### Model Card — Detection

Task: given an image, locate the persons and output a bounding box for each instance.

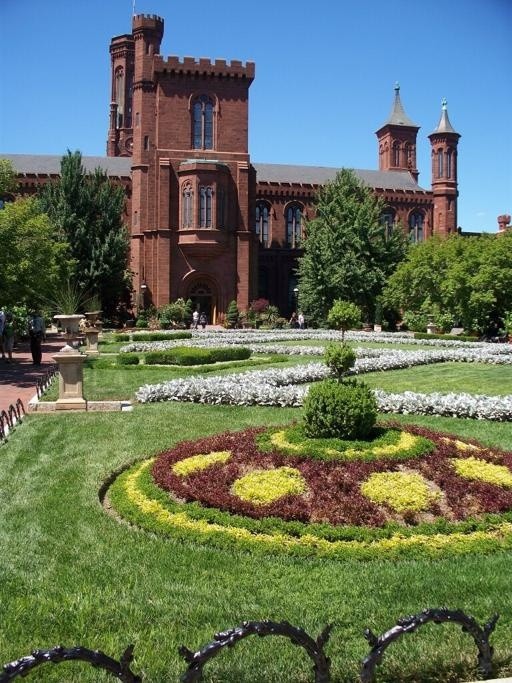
[192,309,199,329]
[200,311,208,329]
[79,317,88,328]
[0,311,7,360]
[4,310,17,363]
[297,312,305,329]
[288,312,297,328]
[28,312,46,367]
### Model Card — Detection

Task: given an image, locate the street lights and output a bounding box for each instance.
[294,287,299,322]
